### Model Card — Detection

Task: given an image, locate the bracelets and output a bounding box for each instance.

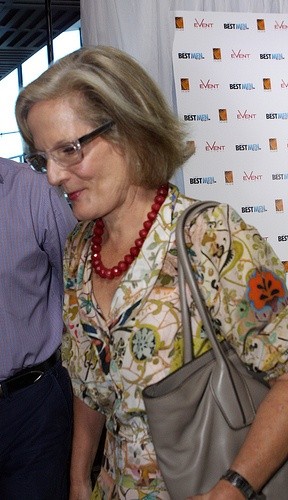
[219,469,257,500]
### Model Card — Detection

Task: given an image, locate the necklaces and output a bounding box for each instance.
[91,185,166,279]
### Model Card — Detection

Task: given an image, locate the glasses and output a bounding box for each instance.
[22,116,122,176]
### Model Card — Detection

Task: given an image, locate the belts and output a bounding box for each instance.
[0,347,63,404]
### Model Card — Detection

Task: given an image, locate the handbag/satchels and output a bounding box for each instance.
[139,196,288,500]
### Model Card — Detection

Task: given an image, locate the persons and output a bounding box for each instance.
[16,45,287,499]
[0,157,80,499]
